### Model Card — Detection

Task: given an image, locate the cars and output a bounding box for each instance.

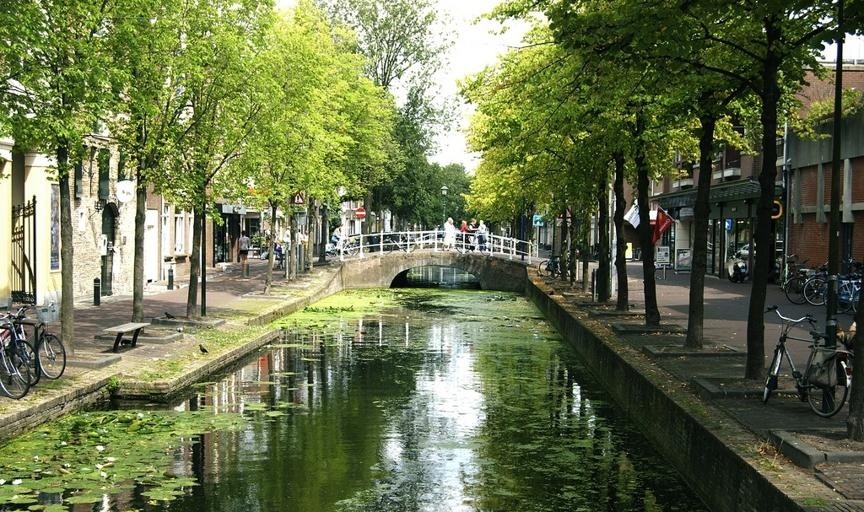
[737,244,757,257]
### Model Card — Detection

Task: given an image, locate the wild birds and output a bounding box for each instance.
[199,344,209,355]
[121,339,131,345]
[164,312,176,319]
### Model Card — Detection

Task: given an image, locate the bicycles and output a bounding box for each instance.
[781,254,861,313]
[383,234,417,252]
[762,305,855,417]
[456,236,490,255]
[539,254,570,279]
[326,238,359,257]
[0,299,66,400]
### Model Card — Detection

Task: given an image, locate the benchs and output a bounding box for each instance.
[102,322,152,353]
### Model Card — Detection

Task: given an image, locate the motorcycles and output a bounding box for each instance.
[727,251,749,283]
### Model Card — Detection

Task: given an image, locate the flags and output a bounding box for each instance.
[652,206,673,245]
[623,199,640,229]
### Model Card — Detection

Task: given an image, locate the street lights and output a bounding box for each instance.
[440,184,448,251]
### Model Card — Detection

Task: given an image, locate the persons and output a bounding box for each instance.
[266,226,346,253]
[238,231,251,267]
[441,217,489,253]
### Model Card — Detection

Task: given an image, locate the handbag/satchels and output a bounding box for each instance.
[806,348,839,389]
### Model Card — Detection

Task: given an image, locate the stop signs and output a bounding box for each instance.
[356,207,366,218]
